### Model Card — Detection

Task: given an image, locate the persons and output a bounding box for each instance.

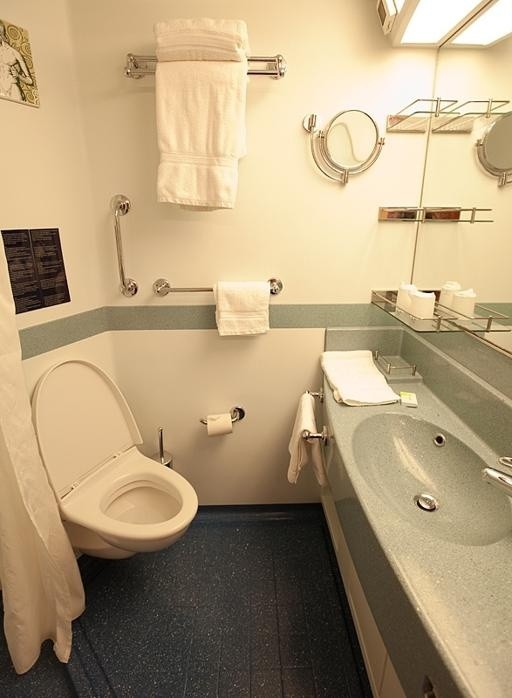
[0,20,34,104]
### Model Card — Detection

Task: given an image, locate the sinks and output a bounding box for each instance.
[353,412,512,548]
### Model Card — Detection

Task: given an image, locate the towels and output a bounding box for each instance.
[286,350,404,489]
[213,279,269,339]
[150,16,246,214]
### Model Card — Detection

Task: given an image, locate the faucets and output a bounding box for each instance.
[481,467,512,495]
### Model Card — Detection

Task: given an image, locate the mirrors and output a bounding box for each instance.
[410,0,512,360]
[320,108,381,179]
[477,110,511,187]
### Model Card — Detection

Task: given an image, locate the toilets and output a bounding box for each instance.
[32,359,198,560]
